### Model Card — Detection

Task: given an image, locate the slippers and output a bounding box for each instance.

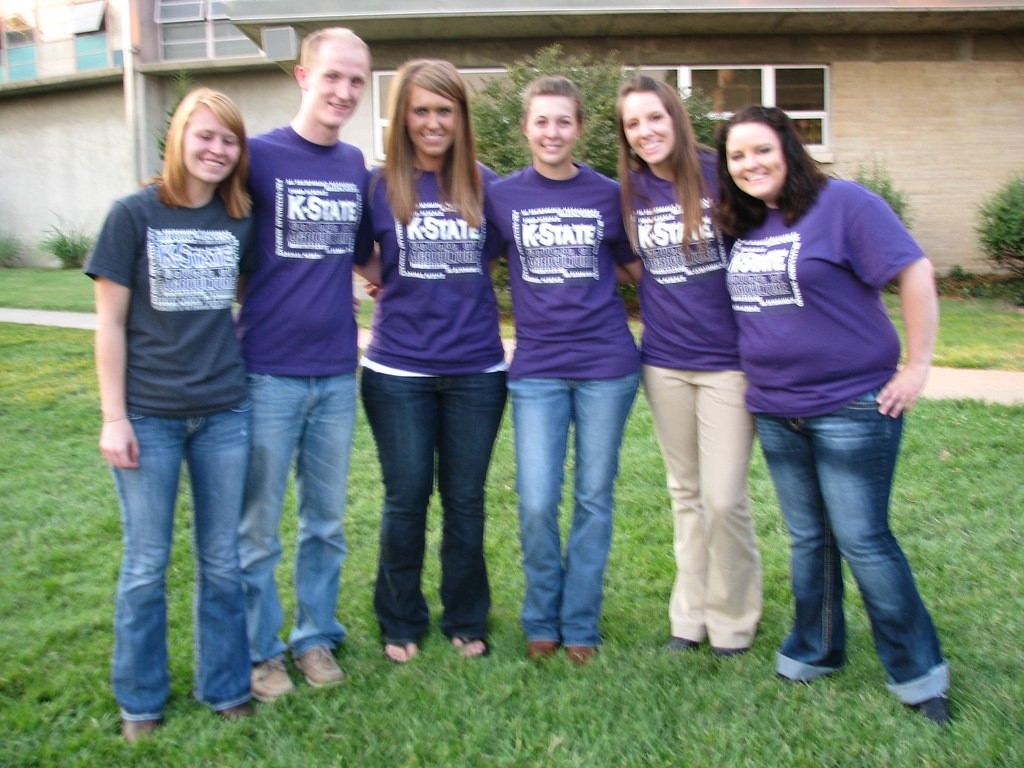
[384,641,421,664]
[449,636,490,656]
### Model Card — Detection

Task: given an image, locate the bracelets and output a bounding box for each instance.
[101,415,129,423]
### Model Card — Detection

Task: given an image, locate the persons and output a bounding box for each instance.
[236,27,385,705]
[82,86,255,741]
[614,77,766,660]
[718,104,954,728]
[358,58,509,665]
[362,75,644,668]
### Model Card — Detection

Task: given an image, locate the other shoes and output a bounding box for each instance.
[294,646,344,688]
[909,697,954,728]
[527,641,559,658]
[566,646,598,664]
[250,657,295,703]
[665,637,698,654]
[219,702,253,722]
[123,720,155,743]
[709,646,749,657]
